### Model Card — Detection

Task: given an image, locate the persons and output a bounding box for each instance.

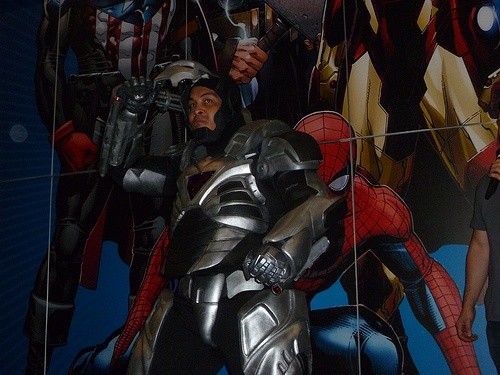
[125,75,346,375]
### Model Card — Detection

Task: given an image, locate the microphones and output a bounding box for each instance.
[485,148,500,200]
